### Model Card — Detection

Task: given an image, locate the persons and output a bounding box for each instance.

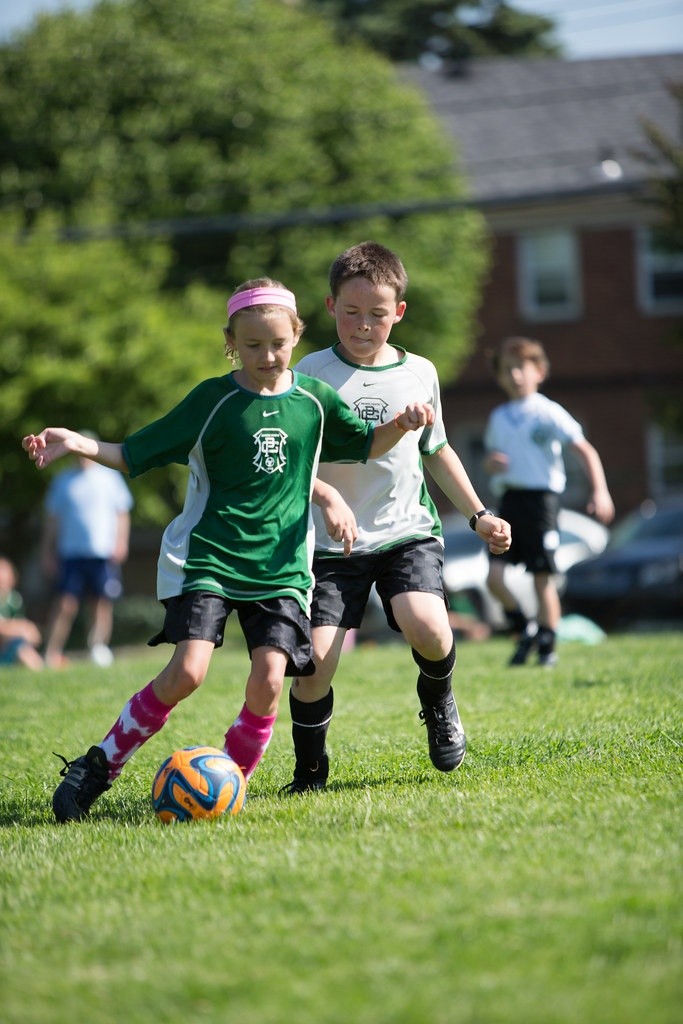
[39,430,134,666]
[476,335,615,667]
[23,279,434,827]
[0,559,46,672]
[280,240,513,800]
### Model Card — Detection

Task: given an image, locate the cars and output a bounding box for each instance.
[555,490,683,644]
[372,505,612,647]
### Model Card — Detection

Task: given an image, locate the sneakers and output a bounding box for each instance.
[416,674,466,772]
[276,751,329,798]
[52,745,113,824]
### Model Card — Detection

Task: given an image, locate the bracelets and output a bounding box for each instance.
[469,509,494,531]
[394,412,409,432]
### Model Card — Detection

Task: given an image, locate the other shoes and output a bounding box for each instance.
[535,650,558,667]
[507,638,533,665]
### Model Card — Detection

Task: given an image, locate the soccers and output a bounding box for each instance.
[151,744,248,825]
